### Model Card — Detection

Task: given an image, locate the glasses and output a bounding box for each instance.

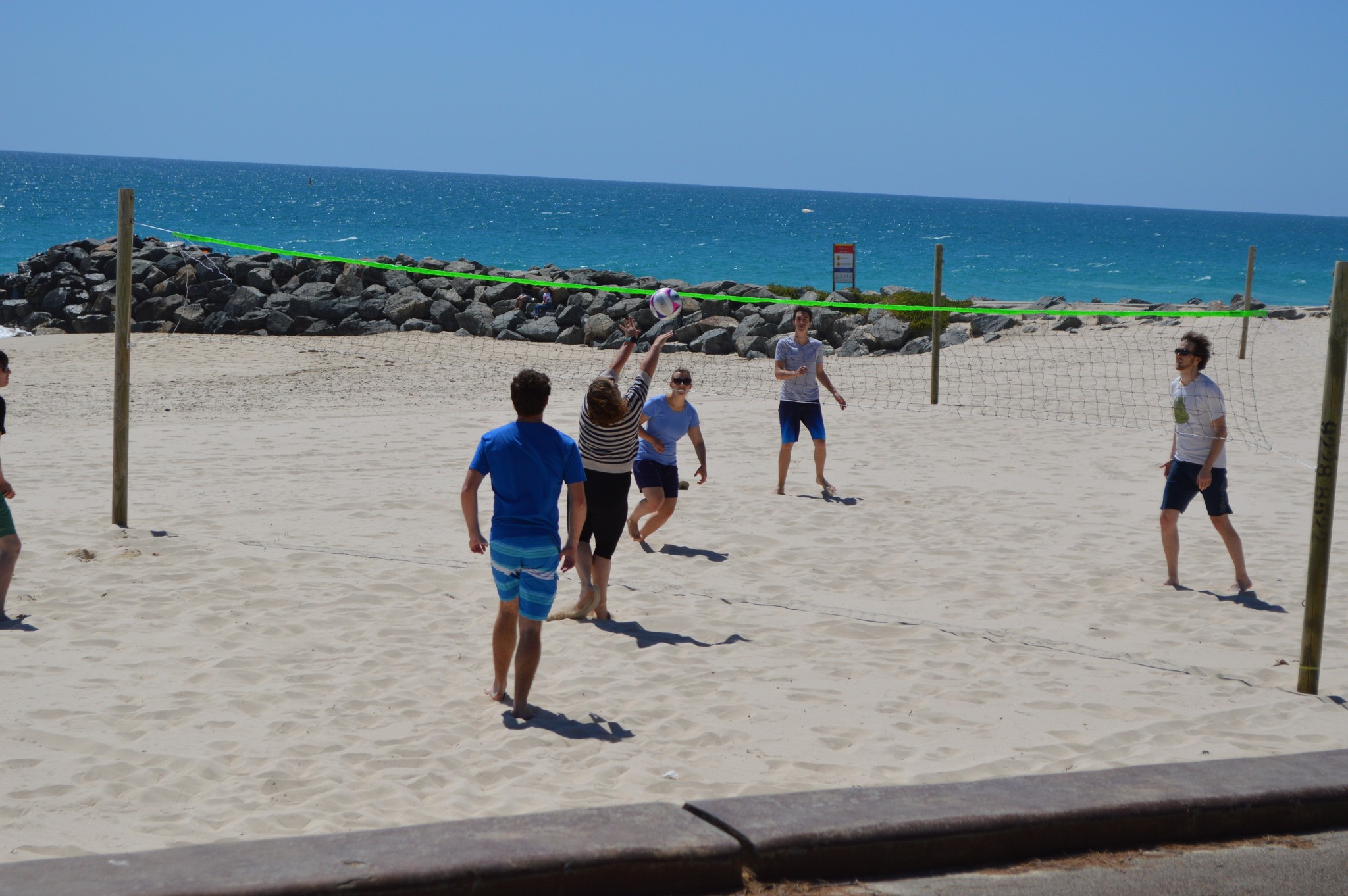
[1175,348,1201,356]
[671,378,692,385]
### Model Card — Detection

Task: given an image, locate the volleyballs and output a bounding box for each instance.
[650,288,682,320]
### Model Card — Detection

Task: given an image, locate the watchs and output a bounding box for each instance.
[625,336,637,344]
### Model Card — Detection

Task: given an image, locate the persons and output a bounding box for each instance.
[1160,331,1253,592]
[566,316,674,621]
[514,278,542,311]
[626,368,707,542]
[0,350,22,622]
[534,286,553,319]
[774,304,846,495]
[460,369,587,720]
